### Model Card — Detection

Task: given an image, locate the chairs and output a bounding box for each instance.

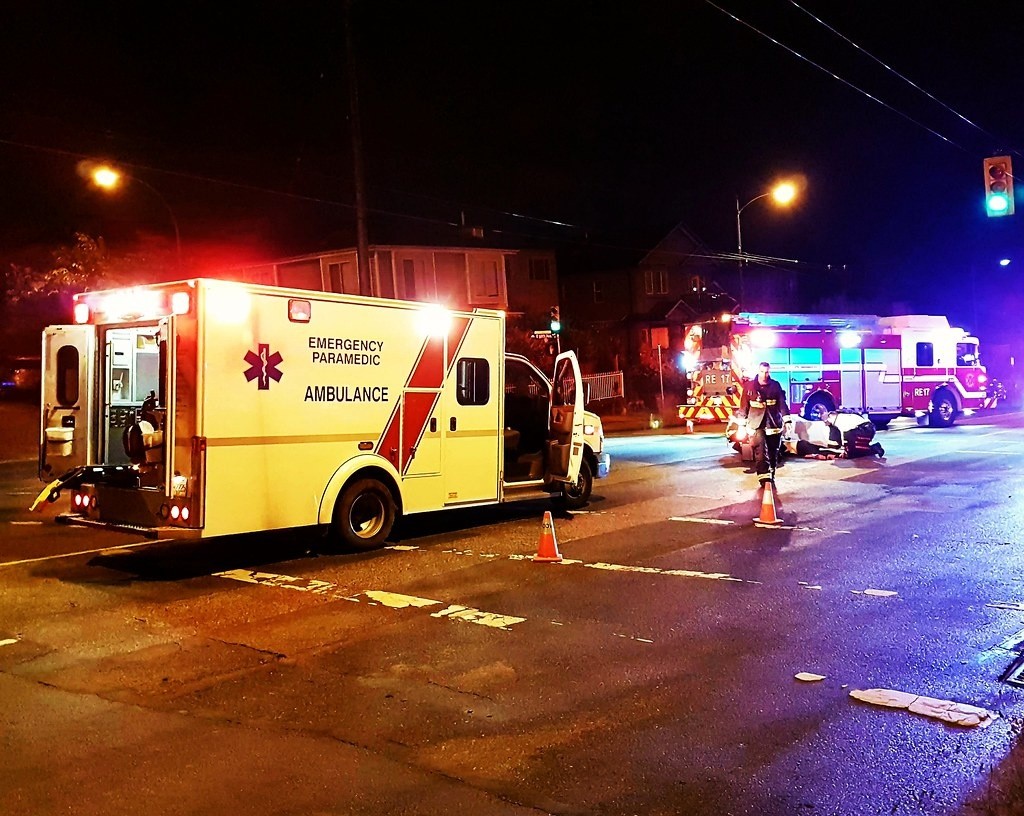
[504,428,520,449]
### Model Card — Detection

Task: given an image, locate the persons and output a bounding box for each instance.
[737,362,794,487]
[725,404,885,458]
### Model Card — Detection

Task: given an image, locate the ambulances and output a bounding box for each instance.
[30,280,611,551]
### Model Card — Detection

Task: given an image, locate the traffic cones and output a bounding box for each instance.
[532,507,565,563]
[753,482,785,526]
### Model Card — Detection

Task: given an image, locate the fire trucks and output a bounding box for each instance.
[675,311,1006,433]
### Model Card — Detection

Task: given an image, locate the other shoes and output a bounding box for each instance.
[761,480,776,490]
[873,442,885,458]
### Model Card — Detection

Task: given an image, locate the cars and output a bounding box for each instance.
[0,344,41,395]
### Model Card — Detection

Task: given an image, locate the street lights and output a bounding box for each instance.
[89,164,184,280]
[735,175,799,310]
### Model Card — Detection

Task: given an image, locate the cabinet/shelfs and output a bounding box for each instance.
[109,327,159,405]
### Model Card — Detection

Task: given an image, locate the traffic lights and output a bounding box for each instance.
[983,155,1015,217]
[548,304,562,333]
